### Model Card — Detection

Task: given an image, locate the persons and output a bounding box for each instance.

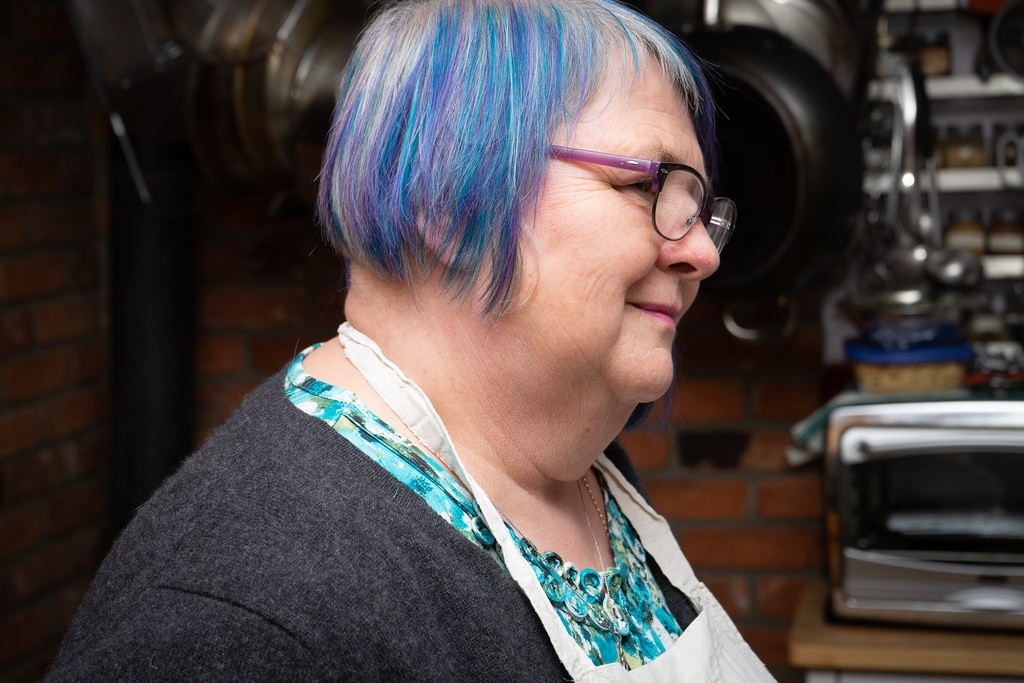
[45,1,786,679]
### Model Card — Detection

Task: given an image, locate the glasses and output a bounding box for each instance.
[542,144,737,258]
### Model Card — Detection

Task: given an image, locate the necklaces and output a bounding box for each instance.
[340,341,634,673]
